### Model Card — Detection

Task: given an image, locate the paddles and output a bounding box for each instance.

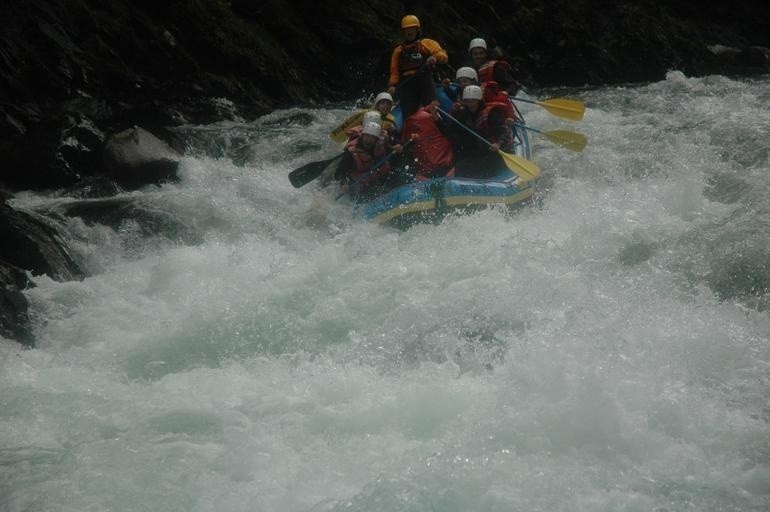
[331,65,427,144]
[435,106,539,180]
[513,121,586,152]
[288,152,345,189]
[509,95,586,120]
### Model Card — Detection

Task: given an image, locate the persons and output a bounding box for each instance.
[332,14,517,201]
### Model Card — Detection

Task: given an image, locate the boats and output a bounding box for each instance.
[353,96,540,232]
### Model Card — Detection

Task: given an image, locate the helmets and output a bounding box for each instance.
[456,67,478,82]
[462,85,482,100]
[362,122,381,138]
[400,15,421,29]
[363,111,381,123]
[375,92,393,106]
[468,38,487,53]
